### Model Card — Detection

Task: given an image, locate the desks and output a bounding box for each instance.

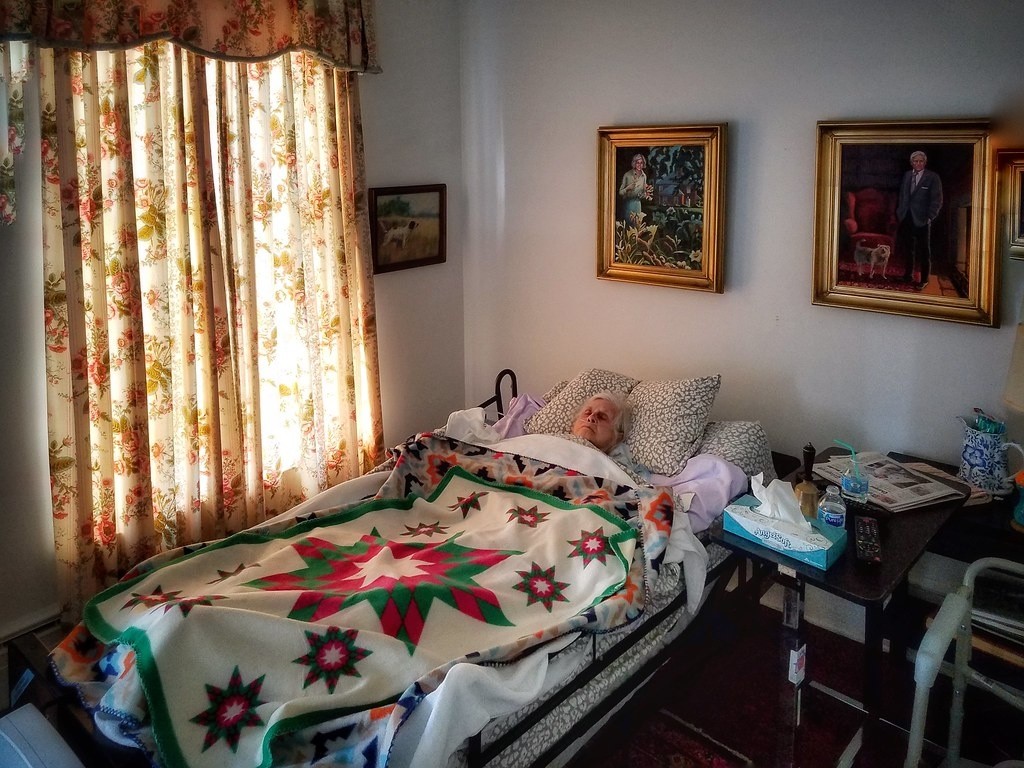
[706,444,973,751]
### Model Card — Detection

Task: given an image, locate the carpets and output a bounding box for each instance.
[584,707,754,768]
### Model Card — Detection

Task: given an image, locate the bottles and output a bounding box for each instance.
[818,485,847,529]
[841,455,870,504]
[795,472,819,519]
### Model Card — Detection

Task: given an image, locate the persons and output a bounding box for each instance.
[569,388,633,459]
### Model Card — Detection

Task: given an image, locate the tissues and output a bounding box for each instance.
[723,472,849,572]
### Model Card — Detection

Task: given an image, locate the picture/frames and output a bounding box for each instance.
[594,122,728,294]
[992,146,1024,260]
[811,117,1000,327]
[367,183,449,276]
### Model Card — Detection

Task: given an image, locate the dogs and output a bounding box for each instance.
[378,220,421,251]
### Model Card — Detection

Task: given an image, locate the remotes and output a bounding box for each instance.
[854,516,883,569]
[815,489,891,520]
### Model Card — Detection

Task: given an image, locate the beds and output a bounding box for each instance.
[7,370,802,767]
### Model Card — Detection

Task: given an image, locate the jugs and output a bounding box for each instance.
[954,415,1024,495]
